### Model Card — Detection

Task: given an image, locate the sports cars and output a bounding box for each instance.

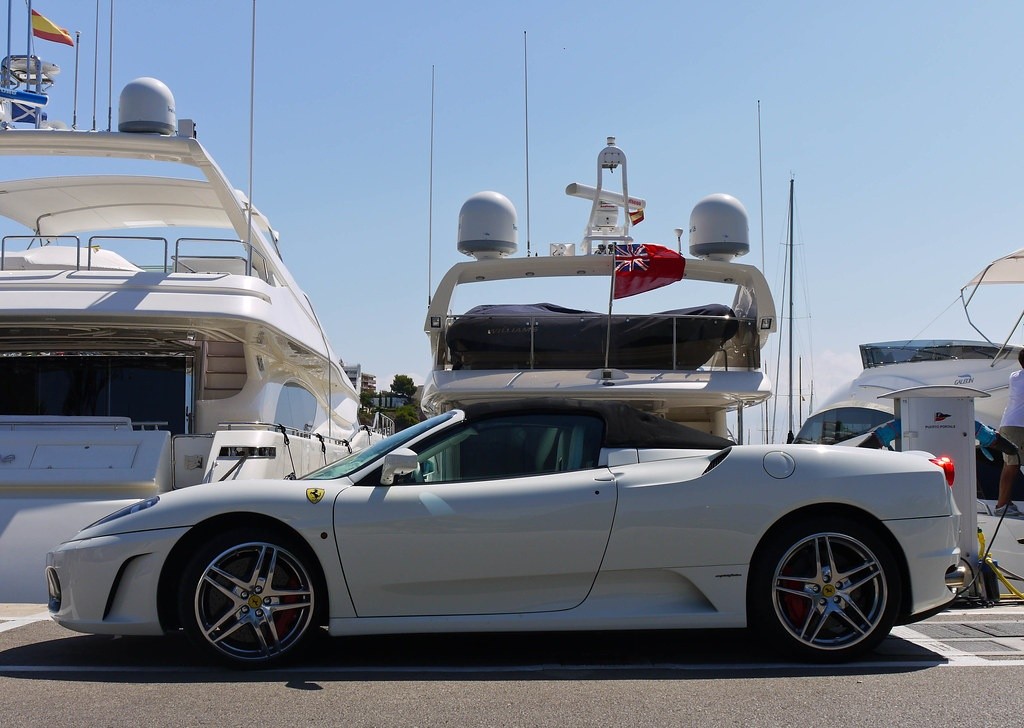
[42,399,969,682]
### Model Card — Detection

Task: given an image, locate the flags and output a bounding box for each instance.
[613,245,650,272]
[613,244,687,299]
[30,7,74,47]
[629,209,646,225]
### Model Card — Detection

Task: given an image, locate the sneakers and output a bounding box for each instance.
[994,505,1021,517]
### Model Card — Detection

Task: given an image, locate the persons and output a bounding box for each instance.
[452,349,477,372]
[992,347,1024,520]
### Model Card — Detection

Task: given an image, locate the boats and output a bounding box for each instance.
[1,1,394,602]
[414,130,785,481]
[793,243,1023,603]
